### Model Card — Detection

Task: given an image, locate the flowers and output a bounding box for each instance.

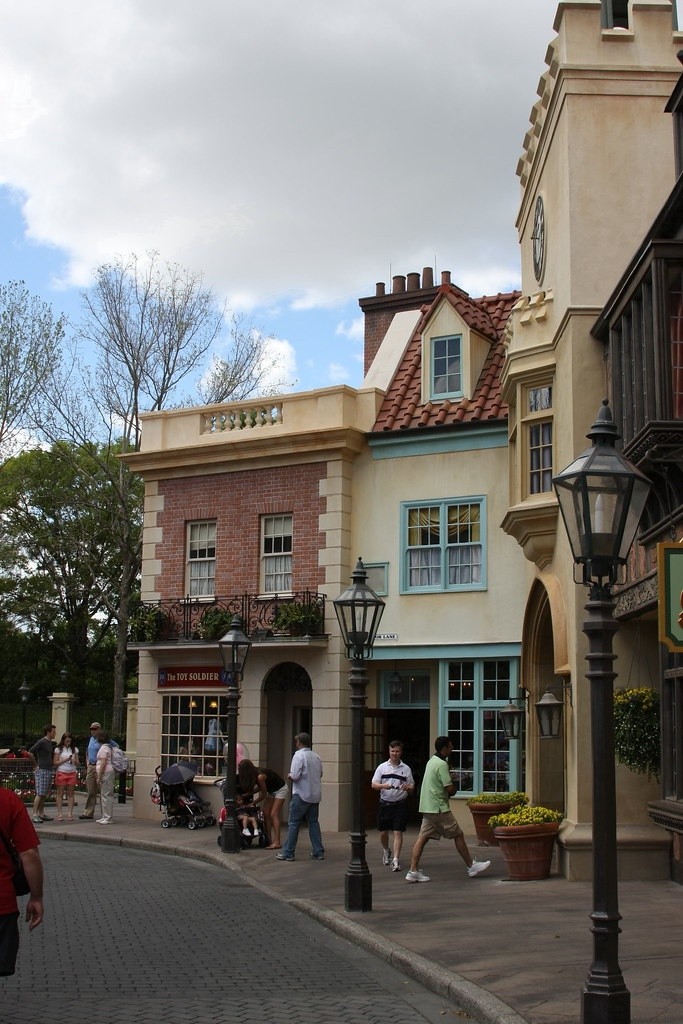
[466,793,529,805]
[613,687,662,786]
[487,803,564,830]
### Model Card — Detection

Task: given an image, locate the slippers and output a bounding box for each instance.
[265,843,282,849]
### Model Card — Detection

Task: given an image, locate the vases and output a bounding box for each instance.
[468,803,515,846]
[493,822,560,882]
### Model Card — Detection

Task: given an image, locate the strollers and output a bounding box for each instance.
[154,761,217,830]
[213,774,270,851]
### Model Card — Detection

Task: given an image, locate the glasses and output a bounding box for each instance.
[390,786,400,790]
[90,728,99,730]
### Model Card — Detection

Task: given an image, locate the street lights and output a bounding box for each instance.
[19,677,30,746]
[216,614,252,853]
[331,557,386,912]
[552,397,654,1024]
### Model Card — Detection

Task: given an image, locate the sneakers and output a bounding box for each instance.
[254,828,258,836]
[382,847,391,865]
[242,828,251,836]
[276,852,294,860]
[95,816,106,823]
[466,860,491,877]
[405,868,431,882]
[389,858,401,871]
[100,816,113,825]
[309,851,324,859]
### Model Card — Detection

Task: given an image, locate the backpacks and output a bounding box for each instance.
[103,744,129,773]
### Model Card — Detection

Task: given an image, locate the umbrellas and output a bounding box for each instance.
[160,761,198,793]
[0,748,10,755]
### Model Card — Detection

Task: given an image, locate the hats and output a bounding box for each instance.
[90,722,101,729]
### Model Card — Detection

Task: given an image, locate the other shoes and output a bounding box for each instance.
[79,814,93,819]
[39,815,54,821]
[68,815,74,821]
[32,816,43,823]
[58,815,64,821]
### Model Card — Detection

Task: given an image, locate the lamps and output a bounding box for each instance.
[533,683,572,739]
[189,700,197,708]
[209,701,218,708]
[499,687,530,740]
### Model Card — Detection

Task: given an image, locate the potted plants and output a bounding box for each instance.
[197,610,244,640]
[271,601,321,637]
[130,609,170,645]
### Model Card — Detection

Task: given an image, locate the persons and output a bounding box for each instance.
[0,746,31,772]
[54,732,79,821]
[276,733,325,861]
[28,725,56,823]
[78,722,120,825]
[238,759,289,850]
[0,786,43,977]
[236,795,259,836]
[405,736,491,883]
[371,741,415,872]
